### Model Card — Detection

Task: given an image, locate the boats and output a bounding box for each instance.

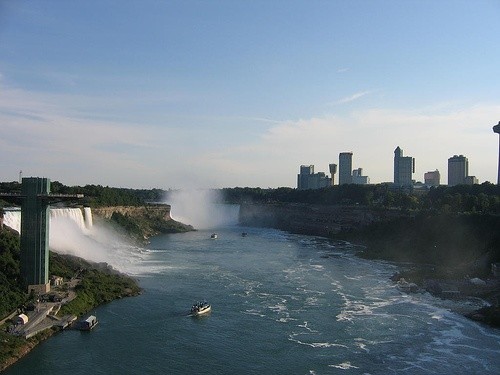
[79,316,99,331]
[191,300,211,316]
[211,233,217,240]
